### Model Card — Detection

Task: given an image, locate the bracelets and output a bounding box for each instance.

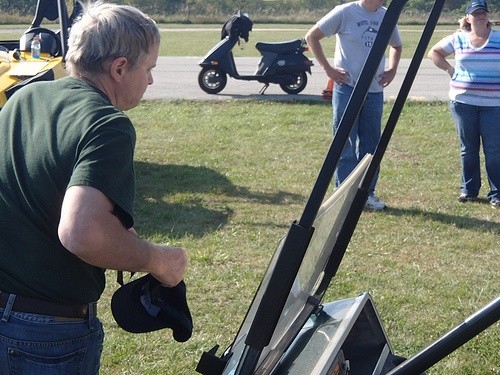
[445,67,453,72]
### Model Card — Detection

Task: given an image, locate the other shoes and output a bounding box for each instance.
[488,198,500,208]
[457,193,479,202]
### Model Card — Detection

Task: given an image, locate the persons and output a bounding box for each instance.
[305,0,403,210]
[0,0,188,375]
[428,0,500,204]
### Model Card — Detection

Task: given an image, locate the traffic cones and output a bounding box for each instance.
[322,77,334,99]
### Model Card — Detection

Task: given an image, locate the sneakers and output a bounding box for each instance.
[365,194,387,210]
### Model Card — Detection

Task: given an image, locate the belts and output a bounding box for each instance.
[0,291,99,320]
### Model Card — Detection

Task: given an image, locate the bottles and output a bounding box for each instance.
[31,36,40,61]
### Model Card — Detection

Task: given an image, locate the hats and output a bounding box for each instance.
[111,272,193,343]
[464,0,490,15]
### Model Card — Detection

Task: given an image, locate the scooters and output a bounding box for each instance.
[197,10,314,95]
